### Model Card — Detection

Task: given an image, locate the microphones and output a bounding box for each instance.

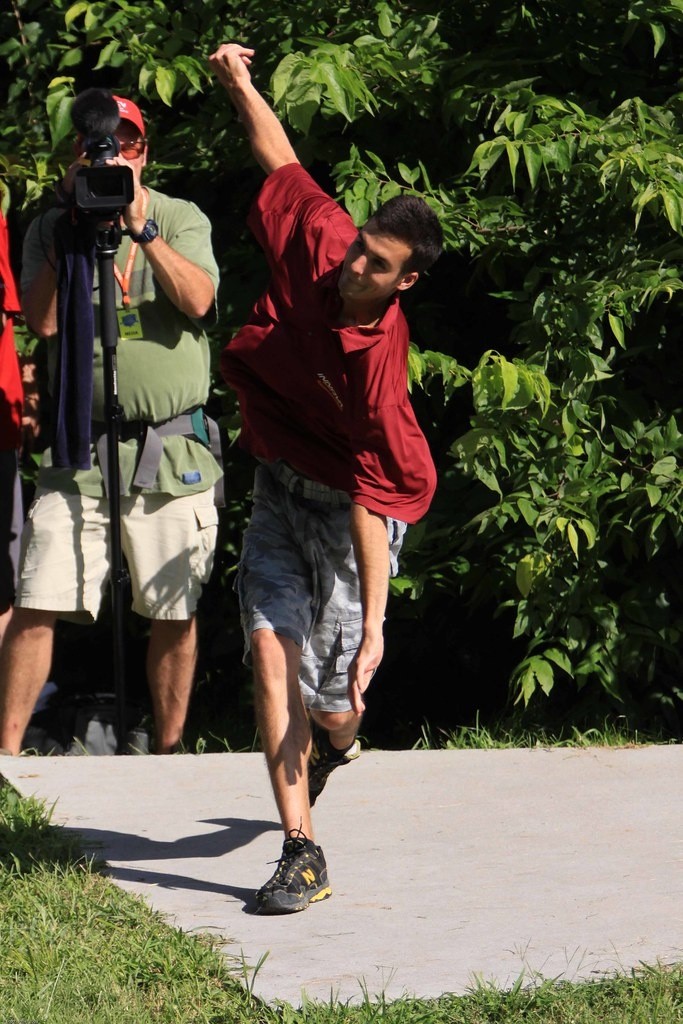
[70,87,121,140]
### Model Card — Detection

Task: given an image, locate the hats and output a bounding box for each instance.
[110,94,145,137]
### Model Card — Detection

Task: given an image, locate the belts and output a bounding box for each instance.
[270,458,353,503]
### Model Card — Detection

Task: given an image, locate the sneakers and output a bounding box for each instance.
[256,838,332,910]
[307,714,360,808]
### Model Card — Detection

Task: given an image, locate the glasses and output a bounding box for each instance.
[118,136,146,161]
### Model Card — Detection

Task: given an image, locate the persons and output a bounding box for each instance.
[204,39,437,917]
[0,84,224,760]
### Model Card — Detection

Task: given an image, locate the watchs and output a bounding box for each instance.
[131,219,158,244]
